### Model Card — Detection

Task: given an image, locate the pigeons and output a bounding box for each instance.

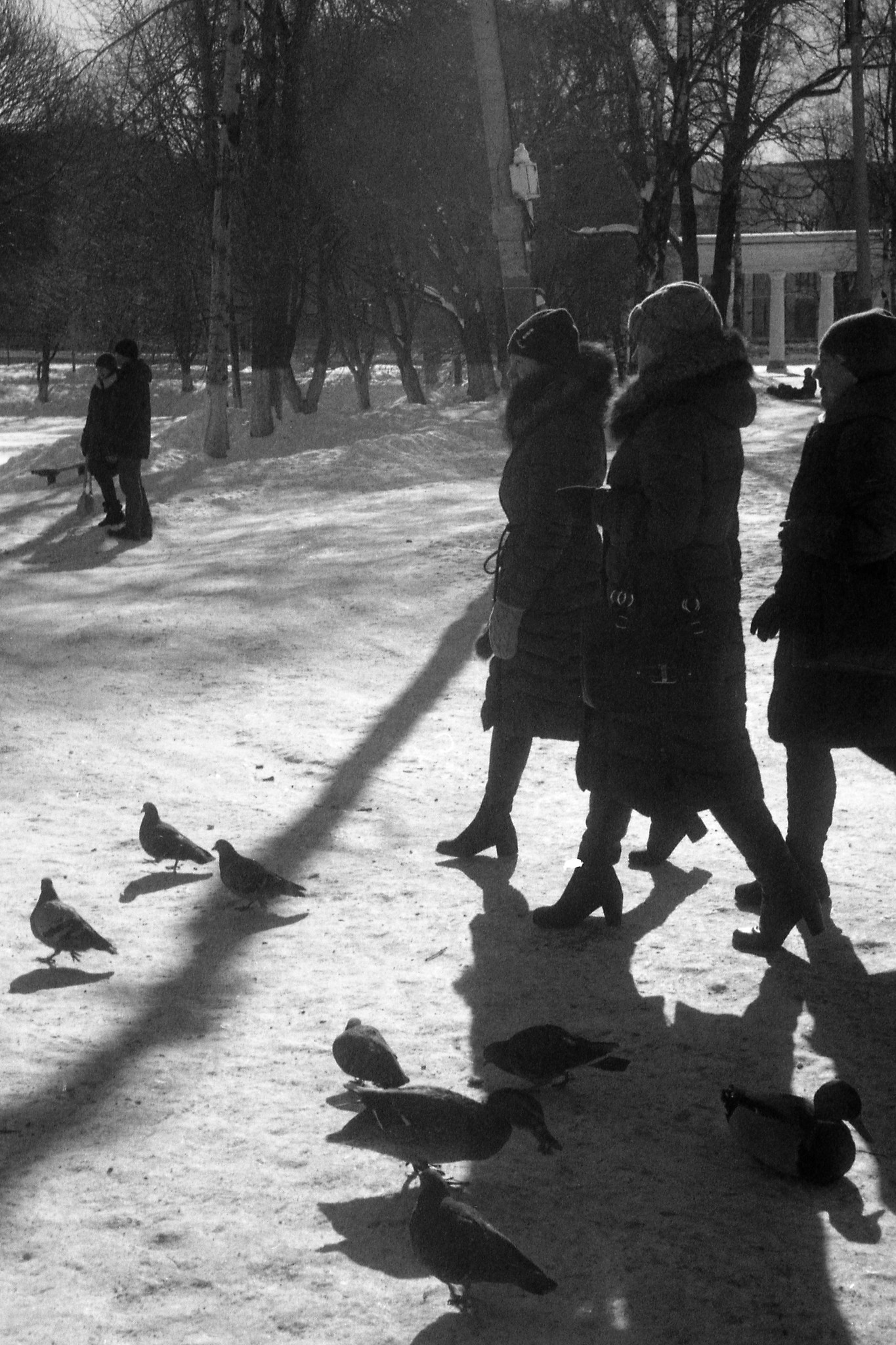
[210,839,309,910]
[409,1169,560,1307]
[31,878,120,965]
[138,801,216,872]
[479,1025,632,1091]
[334,1017,410,1090]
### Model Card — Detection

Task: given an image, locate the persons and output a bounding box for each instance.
[431,304,710,873]
[530,277,827,955]
[796,366,817,401]
[105,338,154,542]
[78,353,127,528]
[729,304,896,954]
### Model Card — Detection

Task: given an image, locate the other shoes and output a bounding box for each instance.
[108,523,153,542]
[735,854,835,903]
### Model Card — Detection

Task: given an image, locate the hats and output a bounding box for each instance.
[817,301,895,384]
[115,339,139,359]
[505,307,579,365]
[627,280,722,356]
[95,354,118,373]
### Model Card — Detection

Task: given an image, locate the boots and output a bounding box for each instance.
[98,501,124,527]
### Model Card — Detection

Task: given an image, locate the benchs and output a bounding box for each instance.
[32,458,88,486]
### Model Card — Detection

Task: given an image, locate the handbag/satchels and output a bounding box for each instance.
[76,491,95,517]
[575,612,713,719]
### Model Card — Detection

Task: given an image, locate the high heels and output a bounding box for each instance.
[628,808,709,867]
[531,866,623,927]
[730,874,826,954]
[433,813,518,860]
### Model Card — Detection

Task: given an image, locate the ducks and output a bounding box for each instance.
[323,1083,564,1185]
[716,1080,876,1189]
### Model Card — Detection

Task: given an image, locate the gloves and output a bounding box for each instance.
[778,514,834,559]
[749,595,788,644]
[555,484,595,520]
[486,600,526,659]
